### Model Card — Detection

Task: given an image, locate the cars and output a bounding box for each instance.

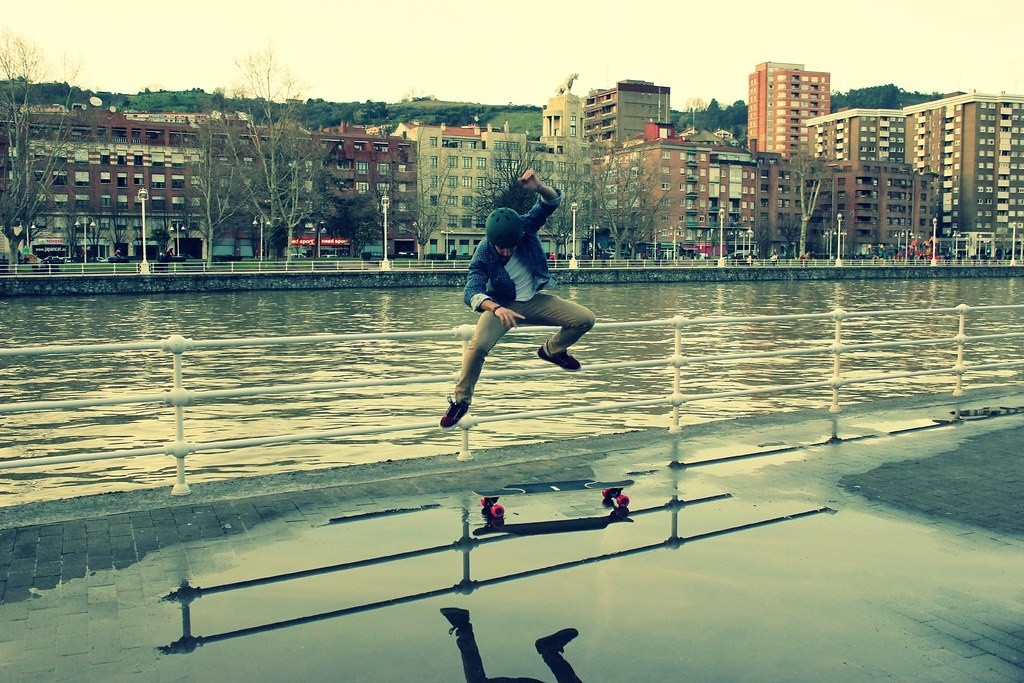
[90,257,107,263]
[291,254,306,258]
[42,255,66,264]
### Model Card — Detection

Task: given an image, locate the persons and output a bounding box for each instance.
[800,255,805,266]
[439,167,595,432]
[166,247,174,260]
[18,250,22,264]
[600,251,607,267]
[772,254,777,267]
[115,248,122,258]
[440,608,583,683]
[550,252,555,268]
[919,253,939,264]
[32,254,38,272]
[909,253,913,264]
[944,252,1001,266]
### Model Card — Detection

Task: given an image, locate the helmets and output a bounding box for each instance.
[485,207,525,245]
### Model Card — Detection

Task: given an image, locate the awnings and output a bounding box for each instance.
[681,244,698,250]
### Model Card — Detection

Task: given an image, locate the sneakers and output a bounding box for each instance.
[440,396,470,431]
[537,345,581,372]
[440,607,470,634]
[535,628,578,654]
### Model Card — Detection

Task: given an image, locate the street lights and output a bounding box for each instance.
[252,214,271,262]
[380,186,390,271]
[841,228,848,260]
[169,220,186,257]
[952,231,961,260]
[441,230,455,260]
[894,230,905,261]
[825,227,837,262]
[310,221,324,258]
[718,207,726,268]
[137,188,150,275]
[746,226,753,258]
[74,214,96,264]
[568,202,579,269]
[729,229,742,266]
[911,232,922,265]
[589,223,600,267]
[19,220,36,263]
[978,233,982,260]
[836,212,843,268]
[901,228,914,262]
[649,227,662,260]
[929,217,938,266]
[669,221,683,264]
[965,235,970,260]
[1008,222,1018,265]
[740,231,749,259]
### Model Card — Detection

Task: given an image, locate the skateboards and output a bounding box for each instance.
[473,478,635,517]
[472,508,635,537]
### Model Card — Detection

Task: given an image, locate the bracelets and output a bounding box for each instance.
[493,306,502,317]
